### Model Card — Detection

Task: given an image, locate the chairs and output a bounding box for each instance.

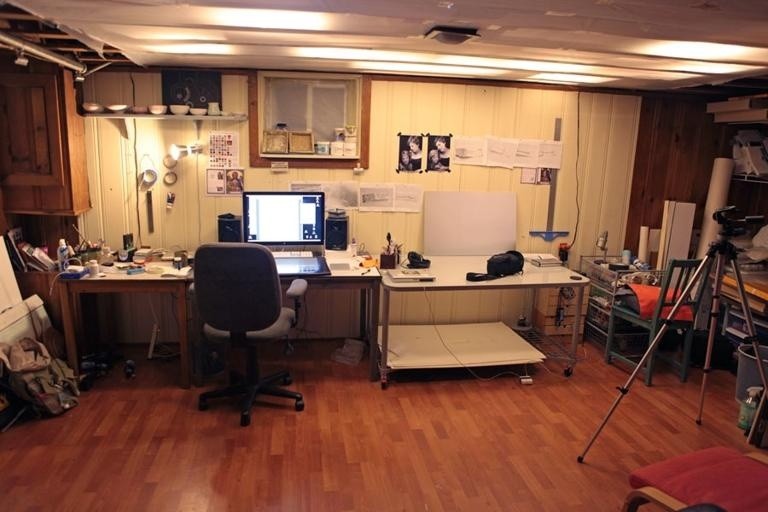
[619,448,768,512]
[188,241,309,425]
[603,257,709,391]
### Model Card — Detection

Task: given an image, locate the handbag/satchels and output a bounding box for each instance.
[465,249,524,283]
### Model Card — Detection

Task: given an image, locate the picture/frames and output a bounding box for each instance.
[287,130,315,154]
[261,129,288,154]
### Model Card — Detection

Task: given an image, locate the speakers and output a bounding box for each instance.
[326,216,349,250]
[218,216,243,242]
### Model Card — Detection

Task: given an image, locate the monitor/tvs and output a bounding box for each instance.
[242,191,326,246]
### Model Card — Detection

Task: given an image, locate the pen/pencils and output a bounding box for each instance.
[361,269,371,276]
[385,244,404,255]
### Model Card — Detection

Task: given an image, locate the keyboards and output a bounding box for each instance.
[271,251,314,258]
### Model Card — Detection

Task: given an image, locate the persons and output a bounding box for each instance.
[428,149,439,170]
[428,136,449,170]
[408,136,422,171]
[399,150,412,170]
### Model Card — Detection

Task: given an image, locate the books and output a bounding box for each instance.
[387,269,436,284]
[4,228,55,273]
[531,257,562,267]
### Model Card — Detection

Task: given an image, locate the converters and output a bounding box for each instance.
[519,375,533,385]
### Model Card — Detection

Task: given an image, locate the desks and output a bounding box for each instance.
[188,249,382,384]
[56,245,193,390]
[370,253,592,389]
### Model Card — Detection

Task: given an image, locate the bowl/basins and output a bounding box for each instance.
[82,102,245,117]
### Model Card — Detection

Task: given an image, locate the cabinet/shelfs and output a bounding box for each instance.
[577,253,670,354]
[1,68,93,216]
[532,268,587,336]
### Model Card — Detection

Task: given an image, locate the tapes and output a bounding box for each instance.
[140,169,158,186]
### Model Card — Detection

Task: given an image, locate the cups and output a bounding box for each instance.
[175,251,188,266]
[132,256,146,264]
[118,250,128,262]
[71,246,101,265]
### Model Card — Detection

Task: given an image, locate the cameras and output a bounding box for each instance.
[711,203,739,226]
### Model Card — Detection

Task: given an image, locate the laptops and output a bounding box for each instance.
[274,257,332,276]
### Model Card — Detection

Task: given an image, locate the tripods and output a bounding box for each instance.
[576,235,768,463]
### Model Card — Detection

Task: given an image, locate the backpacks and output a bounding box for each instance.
[0,336,80,417]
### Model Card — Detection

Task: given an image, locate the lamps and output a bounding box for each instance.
[166,120,204,161]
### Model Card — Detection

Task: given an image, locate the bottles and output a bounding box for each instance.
[173,257,182,269]
[101,247,114,266]
[351,238,356,258]
[89,258,100,277]
[620,249,644,271]
[57,238,68,273]
[275,123,288,131]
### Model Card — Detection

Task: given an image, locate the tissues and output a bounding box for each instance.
[379,239,398,270]
